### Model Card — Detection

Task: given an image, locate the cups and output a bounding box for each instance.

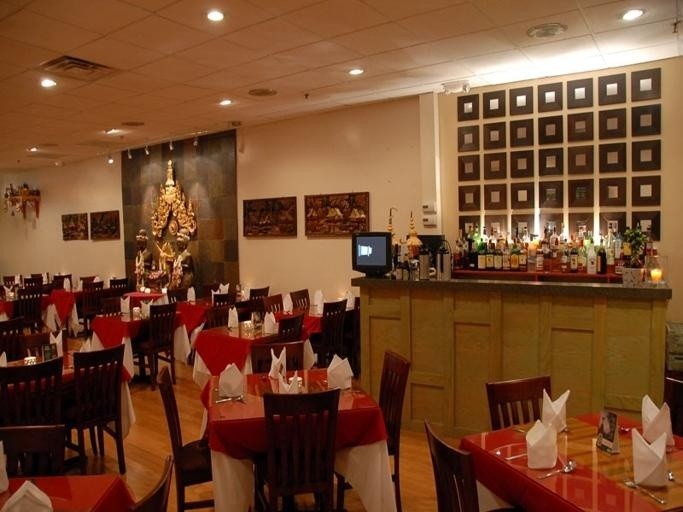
[243,321,251,330]
[24,356,37,366]
[133,307,141,315]
[9,293,14,299]
[644,255,668,285]
[290,376,303,388]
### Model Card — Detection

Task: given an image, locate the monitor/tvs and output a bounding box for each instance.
[351,231,392,278]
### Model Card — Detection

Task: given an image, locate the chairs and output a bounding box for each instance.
[424,420,520,511]
[243,389,334,511]
[130,456,174,512]
[0,272,361,390]
[335,350,410,511]
[1,344,136,476]
[484,375,552,431]
[158,365,214,511]
[664,377,681,437]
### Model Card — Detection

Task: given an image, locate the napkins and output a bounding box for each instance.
[526,419,558,469]
[540,387,570,434]
[2,479,53,511]
[269,347,286,378]
[328,354,353,389]
[639,394,675,451]
[218,362,243,397]
[632,428,669,489]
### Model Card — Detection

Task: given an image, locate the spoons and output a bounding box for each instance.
[502,424,679,507]
[215,395,243,404]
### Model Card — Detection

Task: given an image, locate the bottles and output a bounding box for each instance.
[391,239,449,280]
[622,226,658,269]
[452,224,625,276]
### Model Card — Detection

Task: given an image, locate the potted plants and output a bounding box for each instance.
[616,220,650,284]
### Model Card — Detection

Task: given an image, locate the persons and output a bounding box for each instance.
[162,235,195,297]
[135,230,152,291]
